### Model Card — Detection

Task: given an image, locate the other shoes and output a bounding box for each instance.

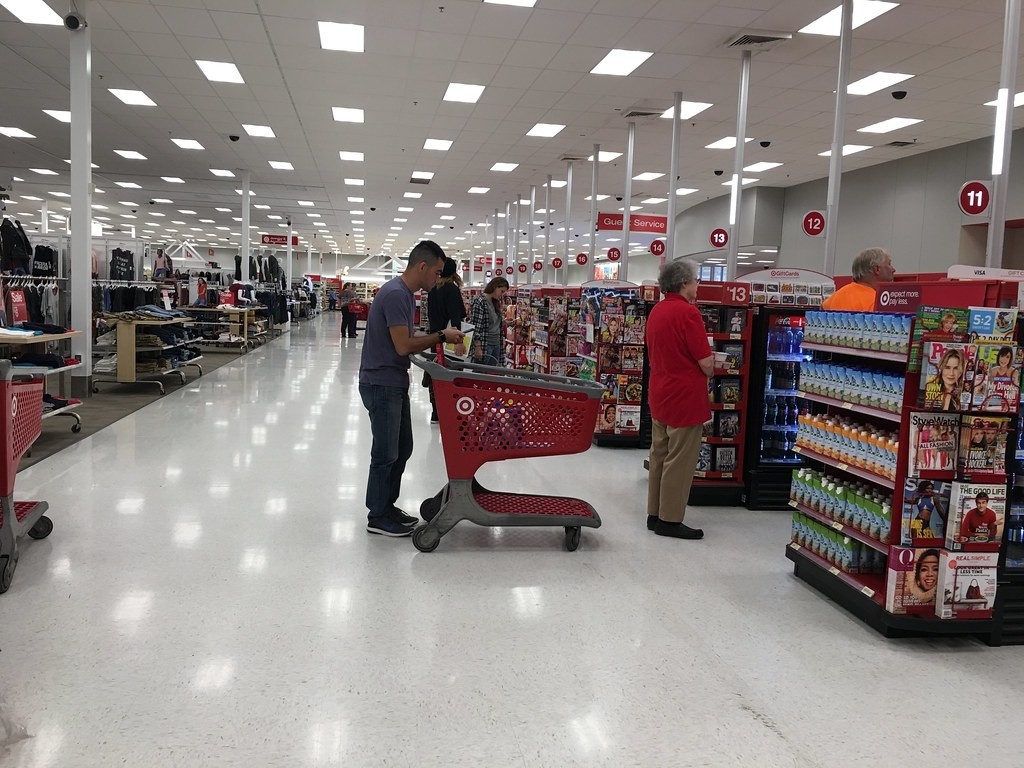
[342,335,346,337]
[431,412,438,423]
[349,335,356,338]
[366,510,415,537]
[655,518,704,539]
[647,515,658,529]
[387,506,418,526]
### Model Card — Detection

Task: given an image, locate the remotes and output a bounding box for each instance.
[346,234,349,236]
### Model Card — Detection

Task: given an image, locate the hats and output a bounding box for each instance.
[440,257,456,277]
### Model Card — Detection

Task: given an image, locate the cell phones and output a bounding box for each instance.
[512,317,520,322]
[462,328,476,334]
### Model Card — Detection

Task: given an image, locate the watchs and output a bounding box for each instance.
[437,331,446,342]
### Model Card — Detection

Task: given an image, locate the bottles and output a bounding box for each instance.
[761,325,802,460]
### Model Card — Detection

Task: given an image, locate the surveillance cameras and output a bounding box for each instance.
[62,12,86,33]
[892,91,907,100]
[286,220,291,226]
[132,210,136,213]
[367,248,370,250]
[371,208,375,211]
[229,136,240,141]
[450,226,454,229]
[616,197,622,201]
[760,141,770,147]
[714,170,723,175]
[523,223,579,238]
[149,201,154,205]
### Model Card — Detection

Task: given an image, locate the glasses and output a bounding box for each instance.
[689,279,701,284]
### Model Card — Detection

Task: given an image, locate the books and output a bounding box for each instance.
[886,306,1024,619]
[503,297,645,436]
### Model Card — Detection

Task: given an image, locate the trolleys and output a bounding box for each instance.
[347,298,371,330]
[0,358,54,593]
[408,349,608,553]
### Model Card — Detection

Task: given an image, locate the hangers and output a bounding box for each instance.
[92,279,157,292]
[0,274,58,290]
[119,243,127,252]
[41,238,51,246]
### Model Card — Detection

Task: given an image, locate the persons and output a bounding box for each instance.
[991,345,1018,394]
[471,277,508,375]
[932,313,957,334]
[421,257,467,425]
[971,422,986,451]
[608,318,619,343]
[365,240,466,537]
[645,261,715,540]
[911,549,939,603]
[338,282,361,338]
[986,421,998,447]
[904,481,945,536]
[960,492,1003,538]
[821,248,896,312]
[938,348,965,410]
[329,288,336,312]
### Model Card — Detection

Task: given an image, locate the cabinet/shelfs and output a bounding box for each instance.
[157,276,323,354]
[356,281,659,449]
[92,315,203,398]
[784,278,1023,647]
[643,280,835,511]
[0,331,84,457]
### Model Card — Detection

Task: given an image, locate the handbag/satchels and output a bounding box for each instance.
[348,300,362,313]
[966,579,980,599]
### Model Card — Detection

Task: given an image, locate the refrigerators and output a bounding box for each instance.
[740,304,820,511]
